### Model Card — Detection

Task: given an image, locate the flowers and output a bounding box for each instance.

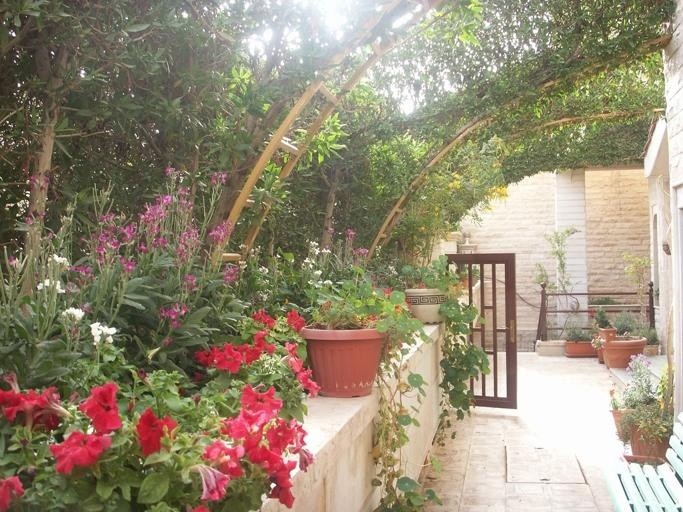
[609,351,674,474]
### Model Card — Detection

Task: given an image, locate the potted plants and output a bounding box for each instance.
[536,225,660,365]
